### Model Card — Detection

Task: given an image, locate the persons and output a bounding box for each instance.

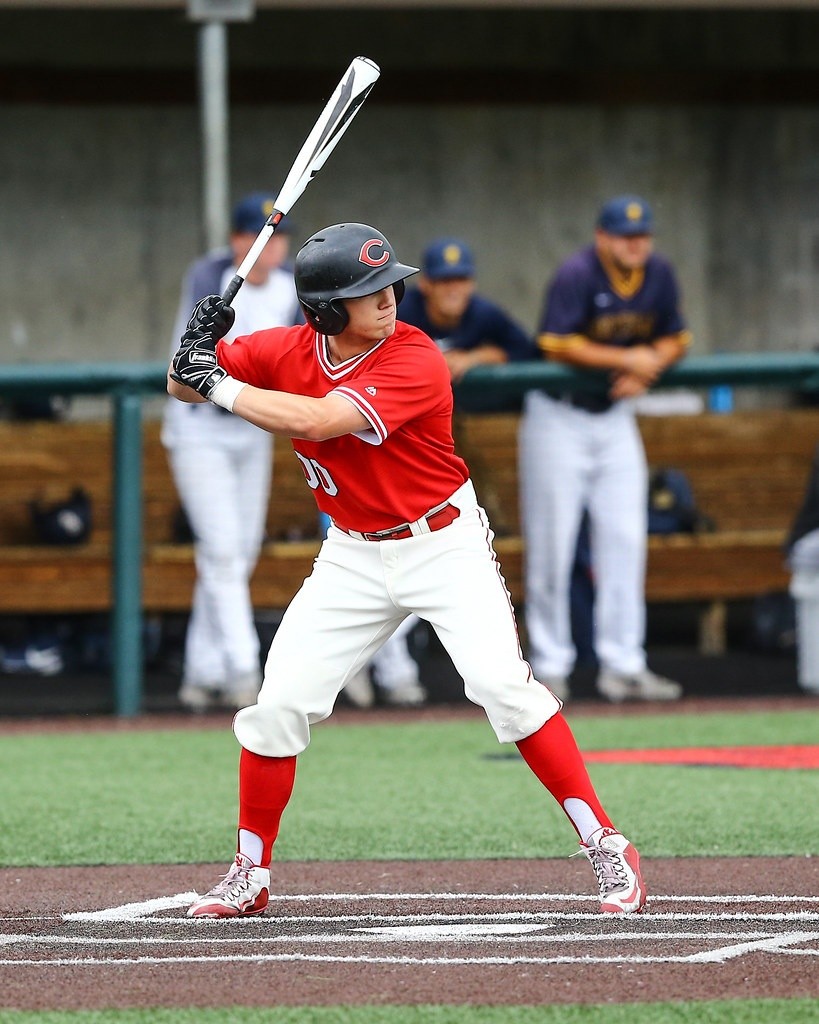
[160,192,306,710]
[166,222,646,919]
[518,192,685,703]
[342,237,537,710]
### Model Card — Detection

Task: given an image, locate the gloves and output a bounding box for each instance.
[185,294,235,345]
[170,330,228,399]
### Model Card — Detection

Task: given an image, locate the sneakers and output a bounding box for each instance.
[187,853,270,918]
[569,826,647,915]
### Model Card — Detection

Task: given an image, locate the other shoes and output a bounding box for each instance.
[221,672,262,708]
[390,680,425,703]
[342,666,373,706]
[542,681,568,702]
[180,686,208,706]
[599,667,680,700]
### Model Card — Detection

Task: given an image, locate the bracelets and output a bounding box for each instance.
[209,374,249,414]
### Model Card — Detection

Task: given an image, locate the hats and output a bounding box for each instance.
[231,193,288,234]
[294,222,420,336]
[422,239,474,278]
[599,196,651,235]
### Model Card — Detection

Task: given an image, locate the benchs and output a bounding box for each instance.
[0,410,818,663]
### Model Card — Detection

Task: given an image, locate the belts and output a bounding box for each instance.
[333,503,460,542]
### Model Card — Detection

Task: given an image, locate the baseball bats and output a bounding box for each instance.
[168,52,384,387]
